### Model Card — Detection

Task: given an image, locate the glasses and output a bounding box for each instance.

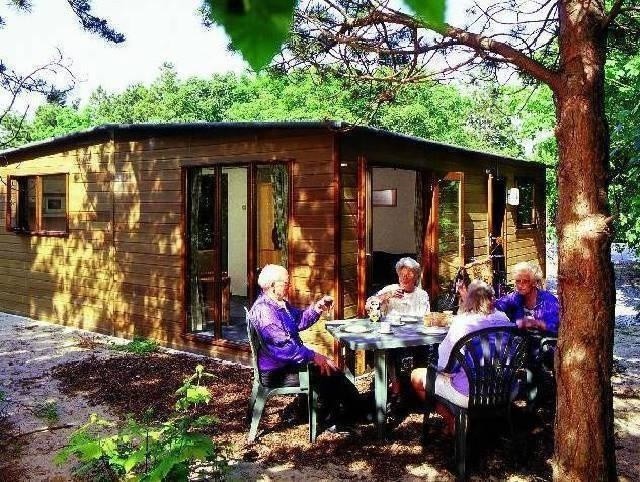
[398,273,415,278]
[516,279,531,284]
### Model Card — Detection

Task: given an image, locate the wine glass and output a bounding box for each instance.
[397,289,404,303]
[324,296,333,316]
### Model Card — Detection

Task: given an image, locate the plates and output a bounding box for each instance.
[340,310,456,335]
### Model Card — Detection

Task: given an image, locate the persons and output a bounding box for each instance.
[410,280,518,437]
[457,265,559,400]
[247,263,393,424]
[365,257,431,404]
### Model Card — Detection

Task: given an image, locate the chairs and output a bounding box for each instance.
[242,306,321,445]
[533,336,555,400]
[423,327,534,475]
